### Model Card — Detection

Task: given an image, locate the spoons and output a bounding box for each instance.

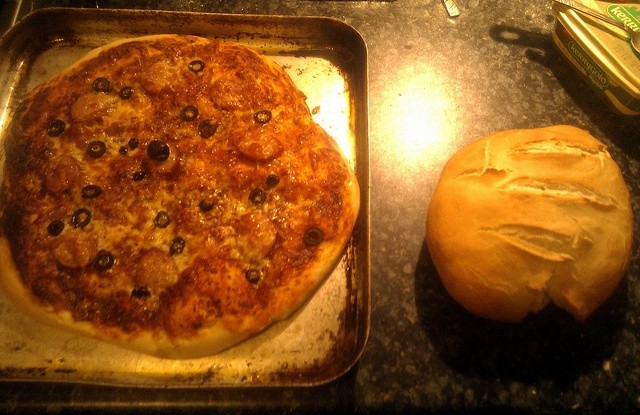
[553,0,640,54]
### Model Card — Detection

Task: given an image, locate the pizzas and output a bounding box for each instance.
[1,34,361,360]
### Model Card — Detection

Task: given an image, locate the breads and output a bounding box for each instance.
[426,125,634,322]
